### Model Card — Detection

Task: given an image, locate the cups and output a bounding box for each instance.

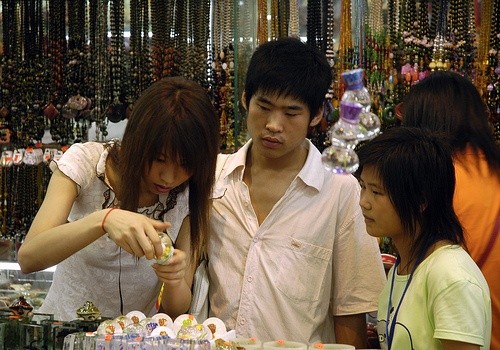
[262,340,307,350]
[309,343,356,349]
[223,337,262,350]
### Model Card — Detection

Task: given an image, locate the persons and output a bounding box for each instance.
[357,126,492,350]
[207,33,386,349]
[17,74,218,323]
[395,69,500,350]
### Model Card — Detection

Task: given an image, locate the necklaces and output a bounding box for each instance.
[0,0,499,139]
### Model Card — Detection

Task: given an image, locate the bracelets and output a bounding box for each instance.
[101,205,116,234]
